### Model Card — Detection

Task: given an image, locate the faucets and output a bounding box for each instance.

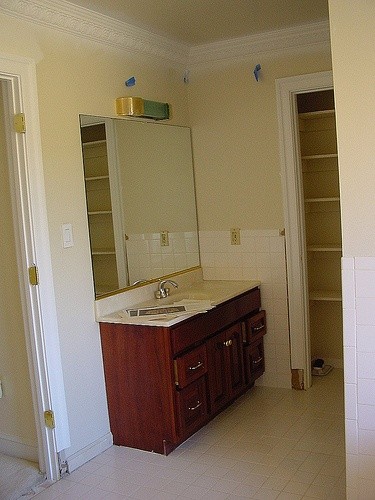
[156,279,178,299]
[130,278,149,285]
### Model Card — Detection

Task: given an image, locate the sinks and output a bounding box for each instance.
[164,299,212,314]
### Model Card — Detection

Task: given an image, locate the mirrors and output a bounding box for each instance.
[77,113,201,300]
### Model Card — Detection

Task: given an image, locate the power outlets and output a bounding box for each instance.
[160,230,168,246]
[230,228,240,245]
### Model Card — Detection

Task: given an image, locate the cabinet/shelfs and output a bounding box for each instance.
[100,312,207,456]
[79,118,129,297]
[241,287,267,390]
[299,111,344,371]
[206,302,247,421]
